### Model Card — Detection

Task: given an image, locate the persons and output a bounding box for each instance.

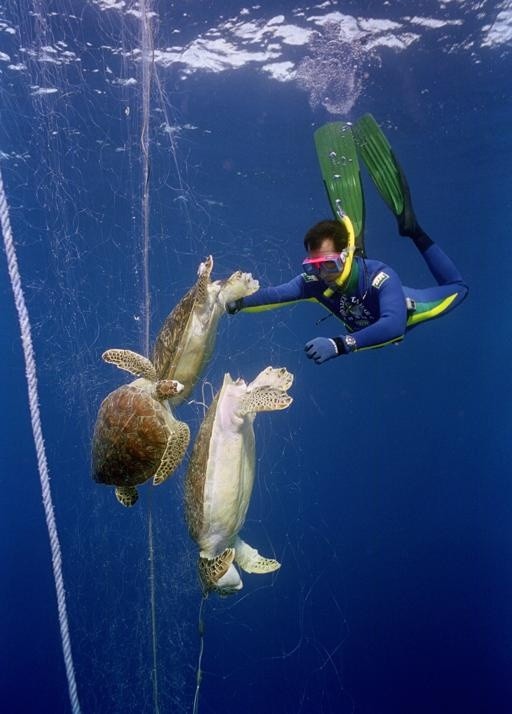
[225,112,470,364]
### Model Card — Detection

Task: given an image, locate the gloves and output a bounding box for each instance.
[302,337,340,364]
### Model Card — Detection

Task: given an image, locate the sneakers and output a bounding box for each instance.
[398,200,413,237]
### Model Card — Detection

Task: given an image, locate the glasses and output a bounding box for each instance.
[302,255,345,276]
